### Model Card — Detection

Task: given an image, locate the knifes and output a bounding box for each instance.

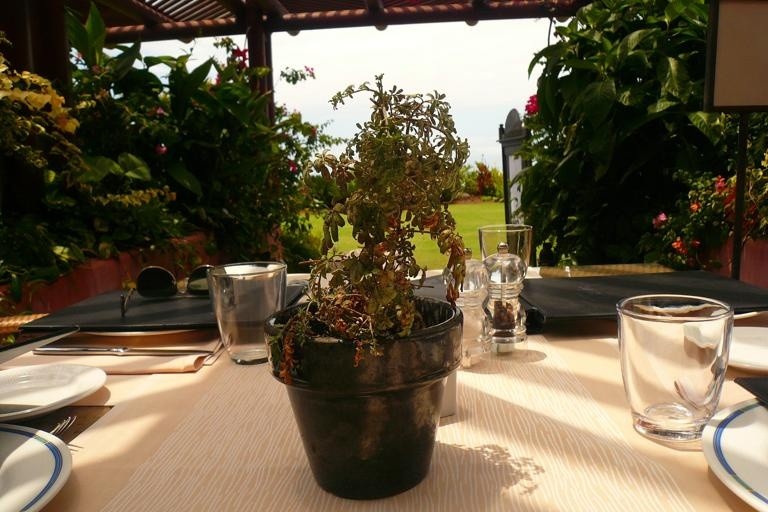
[29,346,215,358]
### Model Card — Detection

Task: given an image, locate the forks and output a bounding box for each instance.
[45,414,80,438]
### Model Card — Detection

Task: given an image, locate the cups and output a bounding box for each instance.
[613,289,736,449]
[477,223,535,260]
[205,260,288,365]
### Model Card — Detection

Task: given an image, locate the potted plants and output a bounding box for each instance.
[262,70,473,502]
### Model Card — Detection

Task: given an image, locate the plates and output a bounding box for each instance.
[721,312,767,374]
[0,362,107,424]
[699,395,768,510]
[0,423,73,511]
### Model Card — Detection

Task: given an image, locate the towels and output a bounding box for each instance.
[0,331,235,375]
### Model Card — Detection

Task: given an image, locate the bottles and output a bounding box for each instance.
[457,241,532,370]
